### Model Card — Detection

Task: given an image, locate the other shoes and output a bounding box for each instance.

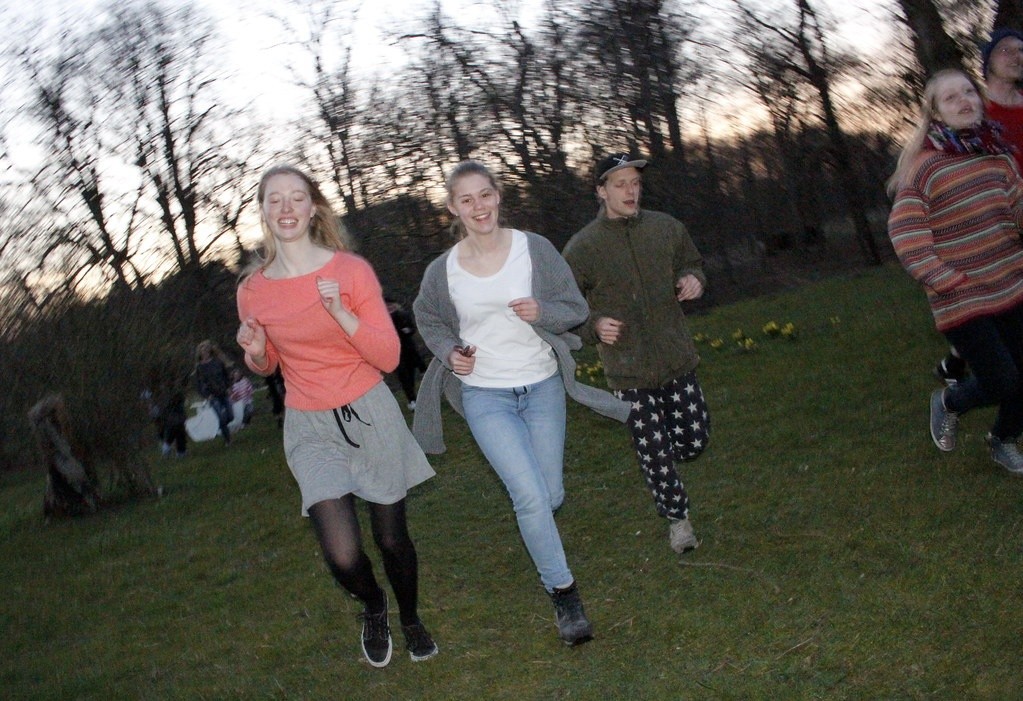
[408,400,416,410]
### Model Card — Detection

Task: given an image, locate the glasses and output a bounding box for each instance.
[994,46,1023,56]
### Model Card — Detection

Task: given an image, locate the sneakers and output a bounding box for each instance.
[361,587,394,667]
[670,520,697,553]
[546,581,596,647]
[930,388,959,451]
[988,432,1023,471]
[401,620,437,662]
[931,358,961,391]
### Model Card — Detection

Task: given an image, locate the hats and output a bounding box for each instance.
[595,152,647,186]
[983,29,1023,73]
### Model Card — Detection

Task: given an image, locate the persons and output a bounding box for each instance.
[411,161,632,648]
[235,166,440,670]
[932,26,1023,388]
[28,334,284,526]
[562,152,712,551]
[889,67,1023,472]
[385,296,428,414]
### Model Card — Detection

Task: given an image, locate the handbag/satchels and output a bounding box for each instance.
[184,405,219,441]
